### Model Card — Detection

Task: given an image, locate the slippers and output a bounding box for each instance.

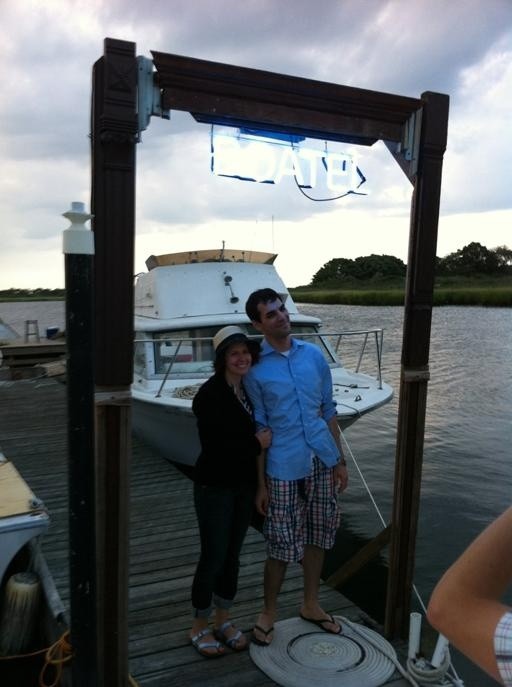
[300,612,342,634]
[251,624,274,646]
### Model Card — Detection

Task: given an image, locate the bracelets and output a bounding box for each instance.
[338,456,347,466]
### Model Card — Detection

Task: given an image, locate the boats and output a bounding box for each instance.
[129,248,393,478]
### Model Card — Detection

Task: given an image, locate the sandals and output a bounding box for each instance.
[191,622,248,658]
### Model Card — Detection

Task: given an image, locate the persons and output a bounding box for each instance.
[425,503,512,686]
[238,286,348,645]
[187,325,273,658]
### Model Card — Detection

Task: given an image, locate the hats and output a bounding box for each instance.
[212,325,260,357]
